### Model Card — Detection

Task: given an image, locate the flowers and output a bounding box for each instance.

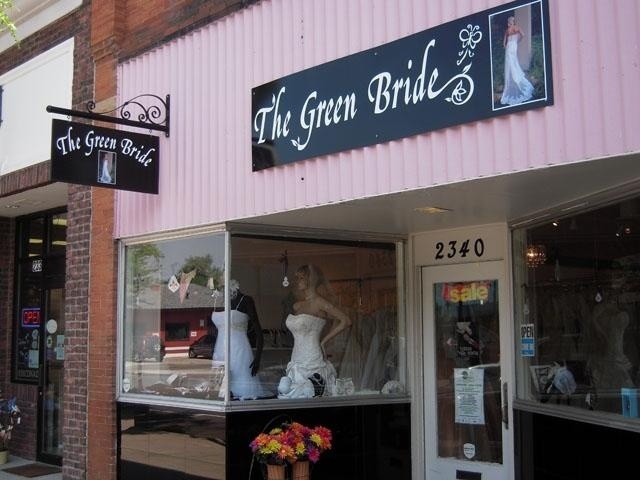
[250,422,331,465]
[0,396,20,452]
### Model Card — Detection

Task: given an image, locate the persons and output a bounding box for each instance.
[276,264,351,397]
[500,16,534,104]
[206,282,264,397]
[99,153,112,183]
[584,287,635,392]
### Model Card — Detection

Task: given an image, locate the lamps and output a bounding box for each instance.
[526,244,547,268]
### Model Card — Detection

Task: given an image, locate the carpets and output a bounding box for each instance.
[2,463,62,478]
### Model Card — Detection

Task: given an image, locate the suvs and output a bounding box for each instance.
[127,334,167,363]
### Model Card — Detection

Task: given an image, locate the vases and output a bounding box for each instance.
[0,449,8,464]
[292,462,309,480]
[267,464,285,480]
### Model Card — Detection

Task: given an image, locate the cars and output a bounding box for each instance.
[188,334,216,358]
